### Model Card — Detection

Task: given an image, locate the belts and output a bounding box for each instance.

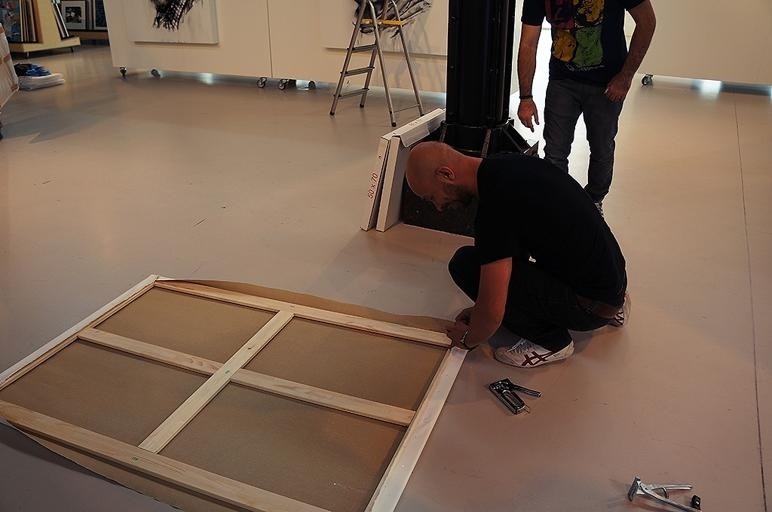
[580,299,619,319]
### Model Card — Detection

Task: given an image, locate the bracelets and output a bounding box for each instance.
[519,96,533,99]
[459,329,478,352]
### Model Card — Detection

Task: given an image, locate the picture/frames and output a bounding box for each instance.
[0,0,107,44]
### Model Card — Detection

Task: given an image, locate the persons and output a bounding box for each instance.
[517,0,657,217]
[406,141,627,366]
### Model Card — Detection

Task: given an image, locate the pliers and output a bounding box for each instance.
[627,477,702,512]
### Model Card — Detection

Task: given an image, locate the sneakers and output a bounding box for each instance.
[495,339,575,368]
[609,293,631,327]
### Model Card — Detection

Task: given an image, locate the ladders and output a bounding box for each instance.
[330,0,424,127]
